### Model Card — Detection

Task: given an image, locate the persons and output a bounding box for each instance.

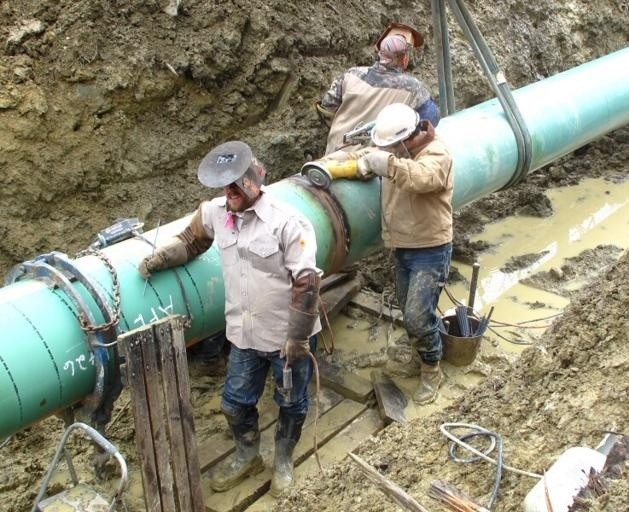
[321,102,455,406]
[138,140,324,499]
[314,36,441,157]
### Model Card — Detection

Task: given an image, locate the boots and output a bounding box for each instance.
[212,416,265,491]
[271,411,305,497]
[413,362,443,405]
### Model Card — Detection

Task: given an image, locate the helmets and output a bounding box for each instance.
[370,104,419,148]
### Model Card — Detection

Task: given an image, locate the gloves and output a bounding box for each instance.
[357,151,394,178]
[139,239,188,278]
[281,307,317,364]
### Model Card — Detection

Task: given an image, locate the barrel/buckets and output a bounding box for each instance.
[439,315,487,366]
